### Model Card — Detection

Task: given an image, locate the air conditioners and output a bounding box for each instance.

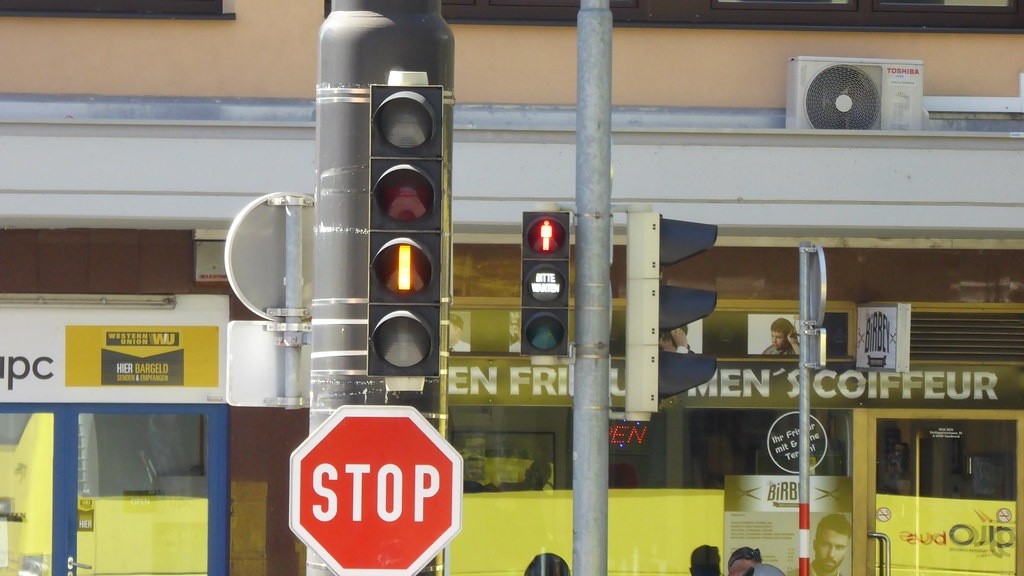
[784,55,927,133]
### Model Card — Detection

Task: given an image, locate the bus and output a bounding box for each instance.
[0,407,1016,574]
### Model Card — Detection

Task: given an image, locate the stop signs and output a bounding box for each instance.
[289,405,464,576]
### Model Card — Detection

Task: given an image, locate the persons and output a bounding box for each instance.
[727,546,788,576]
[762,318,802,355]
[689,544,721,576]
[659,320,695,354]
[809,514,851,576]
[498,459,552,491]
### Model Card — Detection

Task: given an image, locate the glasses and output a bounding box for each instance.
[730,548,760,567]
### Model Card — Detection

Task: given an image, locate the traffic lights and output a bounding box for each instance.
[520,212,570,356]
[366,84,443,376]
[625,207,718,422]
